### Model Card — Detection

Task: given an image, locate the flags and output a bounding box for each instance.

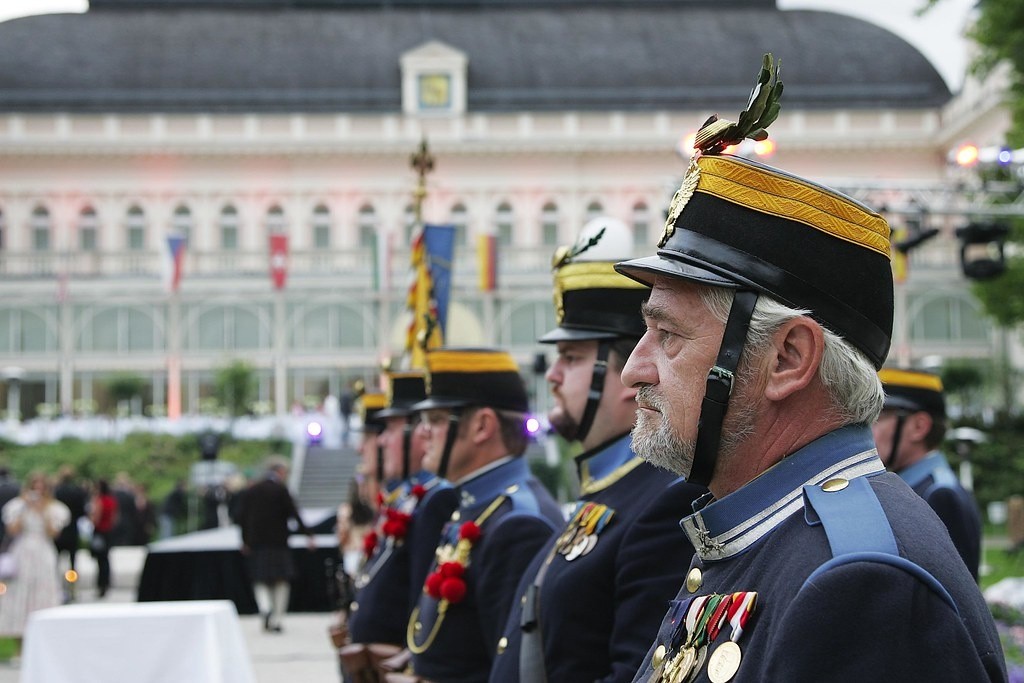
[268,237,288,292]
[479,237,499,286]
[410,224,458,345]
[161,238,184,290]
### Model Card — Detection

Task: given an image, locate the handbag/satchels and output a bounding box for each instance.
[0,552,18,580]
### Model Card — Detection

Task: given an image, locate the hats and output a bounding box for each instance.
[612,151,895,372]
[536,251,655,343]
[375,372,431,419]
[353,393,393,436]
[408,346,529,419]
[875,367,944,414]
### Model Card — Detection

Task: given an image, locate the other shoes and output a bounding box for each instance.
[264,610,282,633]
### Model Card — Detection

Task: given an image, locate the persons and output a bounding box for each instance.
[621,156,1012,681]
[311,350,563,683]
[1,453,310,650]
[870,363,982,585]
[491,253,754,680]
